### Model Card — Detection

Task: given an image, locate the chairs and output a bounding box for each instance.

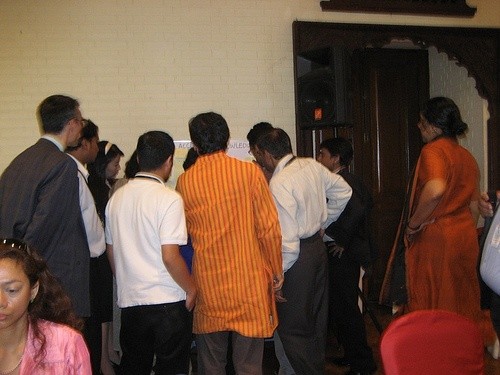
[379,309,485,375]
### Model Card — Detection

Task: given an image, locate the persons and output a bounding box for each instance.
[0,95,500,375]
[175,112,284,375]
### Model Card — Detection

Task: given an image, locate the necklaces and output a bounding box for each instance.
[78,169,87,184]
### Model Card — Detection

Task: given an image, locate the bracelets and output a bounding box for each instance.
[408,224,421,230]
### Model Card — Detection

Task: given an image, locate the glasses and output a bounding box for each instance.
[0,238,27,253]
[75,117,88,127]
[248,147,254,155]
